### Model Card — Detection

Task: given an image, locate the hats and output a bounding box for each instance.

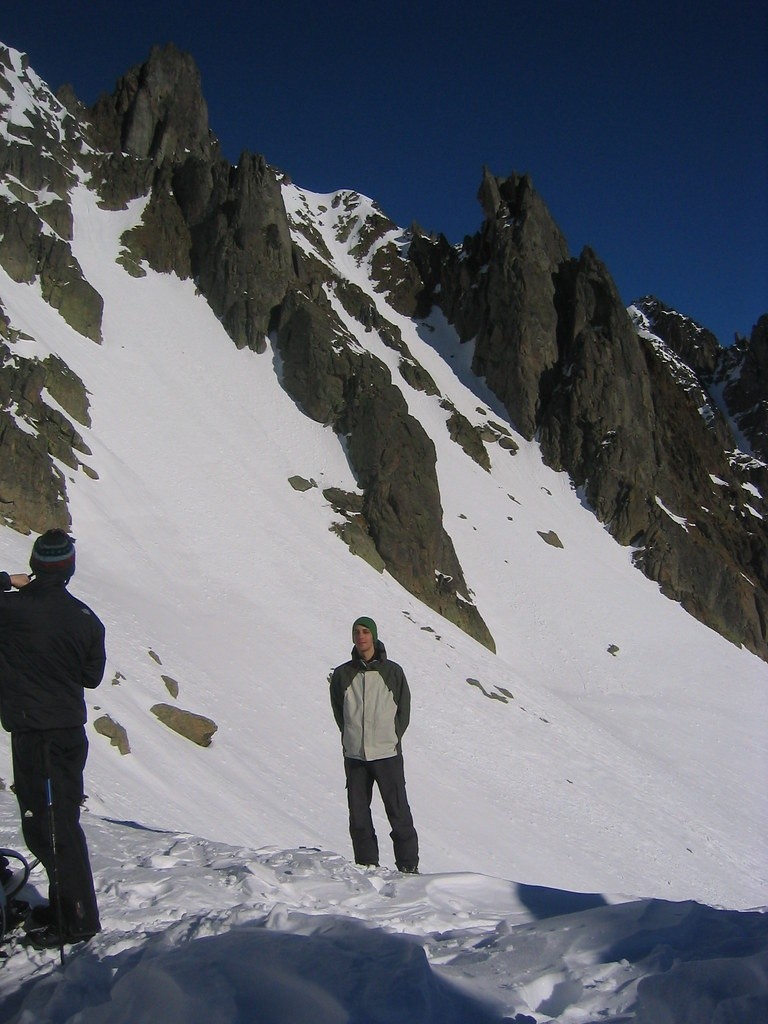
[29,528,76,579]
[352,617,377,643]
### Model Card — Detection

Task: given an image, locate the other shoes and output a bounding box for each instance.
[396,863,419,875]
[31,905,54,924]
[26,923,83,950]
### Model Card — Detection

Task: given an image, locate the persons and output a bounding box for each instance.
[329,617,419,874]
[0,527,107,950]
